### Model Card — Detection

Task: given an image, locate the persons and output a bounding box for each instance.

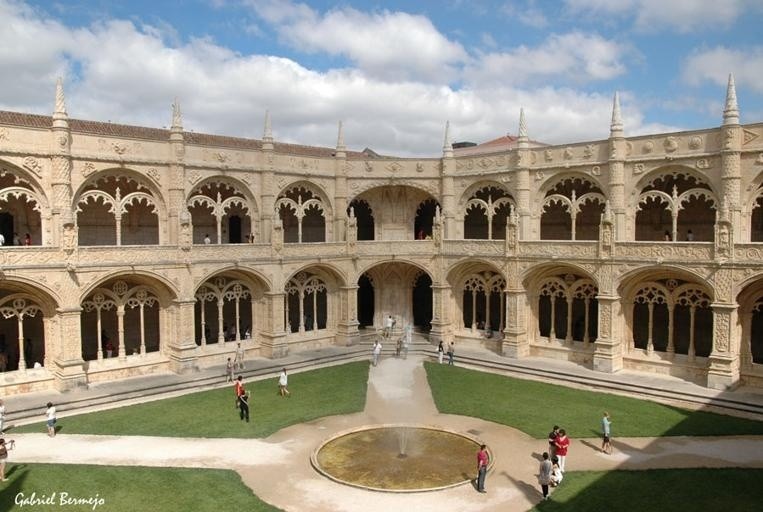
[0,234,5,245]
[193,317,251,346]
[553,427,569,474]
[23,232,31,245]
[418,227,424,240]
[537,451,552,502]
[663,230,671,241]
[547,426,559,461]
[424,232,431,240]
[0,332,33,372]
[284,309,326,333]
[685,229,693,241]
[101,329,110,356]
[233,342,247,371]
[600,410,612,454]
[44,401,56,437]
[463,316,505,330]
[203,233,211,244]
[82,332,90,362]
[277,367,290,399]
[476,444,487,493]
[369,313,457,368]
[225,357,235,384]
[0,438,15,482]
[236,375,244,408]
[0,399,7,437]
[105,341,114,358]
[548,454,563,488]
[239,390,252,422]
[13,232,20,245]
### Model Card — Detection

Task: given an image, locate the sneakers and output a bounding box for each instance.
[478,489,488,494]
